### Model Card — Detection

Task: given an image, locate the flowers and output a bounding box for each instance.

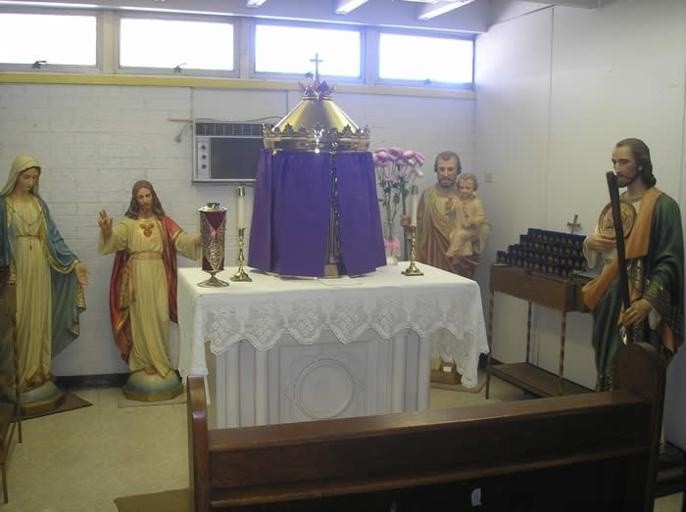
[369,148,426,220]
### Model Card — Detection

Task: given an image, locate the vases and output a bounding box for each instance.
[385,222,400,264]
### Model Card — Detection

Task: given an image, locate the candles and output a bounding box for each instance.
[408,181,420,227]
[237,185,247,231]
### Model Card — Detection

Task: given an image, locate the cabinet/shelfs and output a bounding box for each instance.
[483,262,600,402]
[0,277,24,504]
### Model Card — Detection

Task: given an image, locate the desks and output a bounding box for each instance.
[173,259,487,427]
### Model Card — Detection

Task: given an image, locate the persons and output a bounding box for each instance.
[96,180,201,375]
[415,151,476,282]
[446,172,491,258]
[0,153,91,391]
[580,137,685,394]
